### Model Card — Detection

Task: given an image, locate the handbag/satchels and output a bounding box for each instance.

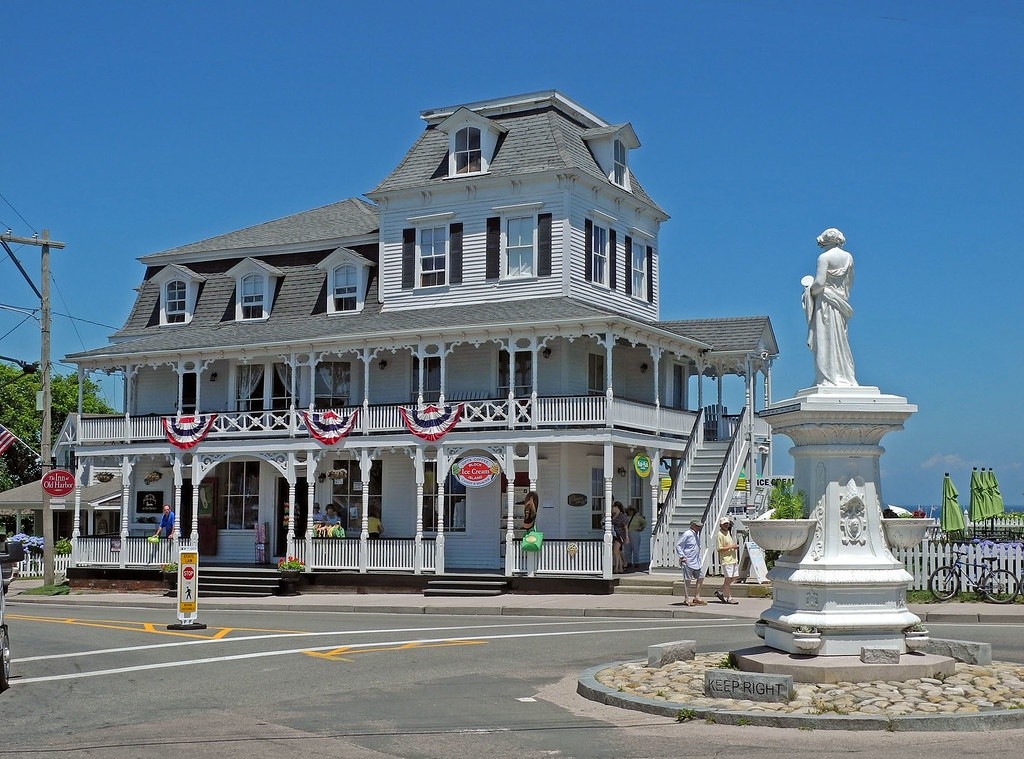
[148,535,159,543]
[521,521,543,551]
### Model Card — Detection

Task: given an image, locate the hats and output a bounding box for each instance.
[690,518,703,527]
[625,505,638,515]
[720,517,730,525]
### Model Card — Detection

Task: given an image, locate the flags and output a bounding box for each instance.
[0,424,17,456]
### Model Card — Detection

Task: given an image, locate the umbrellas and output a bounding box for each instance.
[980,468,995,539]
[941,472,965,546]
[968,466,985,539]
[987,467,1004,534]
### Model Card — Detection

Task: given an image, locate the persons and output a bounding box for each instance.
[801,228,860,388]
[368,513,384,539]
[625,505,646,568]
[714,517,739,605]
[313,503,324,521]
[153,504,175,539]
[322,504,340,525]
[675,519,707,606]
[612,501,629,574]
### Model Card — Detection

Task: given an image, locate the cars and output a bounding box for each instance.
[0,533,25,694]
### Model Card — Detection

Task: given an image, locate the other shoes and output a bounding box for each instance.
[714,590,726,602]
[683,600,695,606]
[727,599,738,604]
[623,563,629,571]
[693,599,706,604]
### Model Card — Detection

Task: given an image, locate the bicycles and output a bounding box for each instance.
[929,550,1020,604]
[1020,575,1024,598]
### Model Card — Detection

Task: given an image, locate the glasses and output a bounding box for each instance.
[723,522,729,525]
[314,506,319,509]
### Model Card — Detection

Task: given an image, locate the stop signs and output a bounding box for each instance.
[183,567,194,580]
